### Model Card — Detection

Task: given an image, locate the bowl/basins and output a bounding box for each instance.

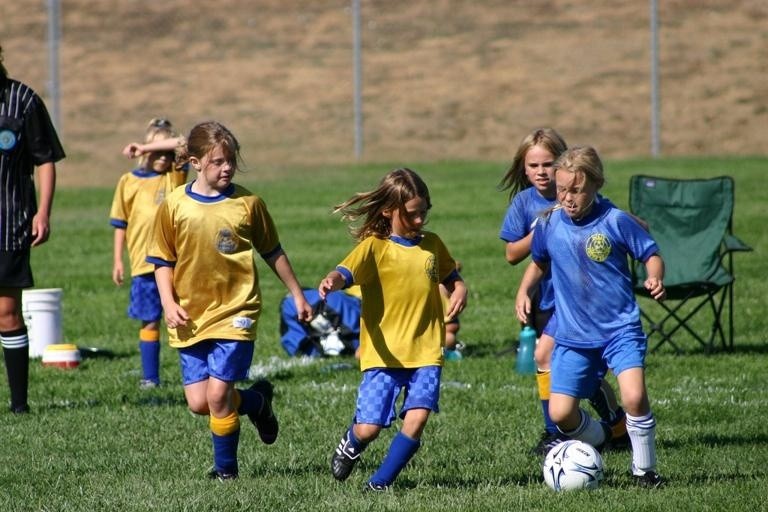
[39,344,86,369]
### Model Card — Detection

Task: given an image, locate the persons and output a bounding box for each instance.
[319,169,468,495]
[145,120,314,481]
[515,146,666,486]
[494,128,649,453]
[109,119,190,391]
[0,45,66,412]
[439,260,467,351]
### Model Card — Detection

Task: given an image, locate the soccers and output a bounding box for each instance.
[543,440,604,493]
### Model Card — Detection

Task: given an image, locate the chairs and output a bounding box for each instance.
[629,175,753,353]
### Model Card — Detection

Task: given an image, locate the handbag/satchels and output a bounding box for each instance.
[277,285,363,360]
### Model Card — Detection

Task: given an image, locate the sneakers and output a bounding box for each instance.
[529,408,632,464]
[626,469,664,491]
[245,378,280,445]
[207,468,239,484]
[362,481,392,496]
[331,428,366,483]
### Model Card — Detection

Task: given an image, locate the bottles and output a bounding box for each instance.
[515,325,536,372]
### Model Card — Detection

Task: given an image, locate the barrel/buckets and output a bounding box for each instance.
[20,288,63,357]
[43,344,80,368]
